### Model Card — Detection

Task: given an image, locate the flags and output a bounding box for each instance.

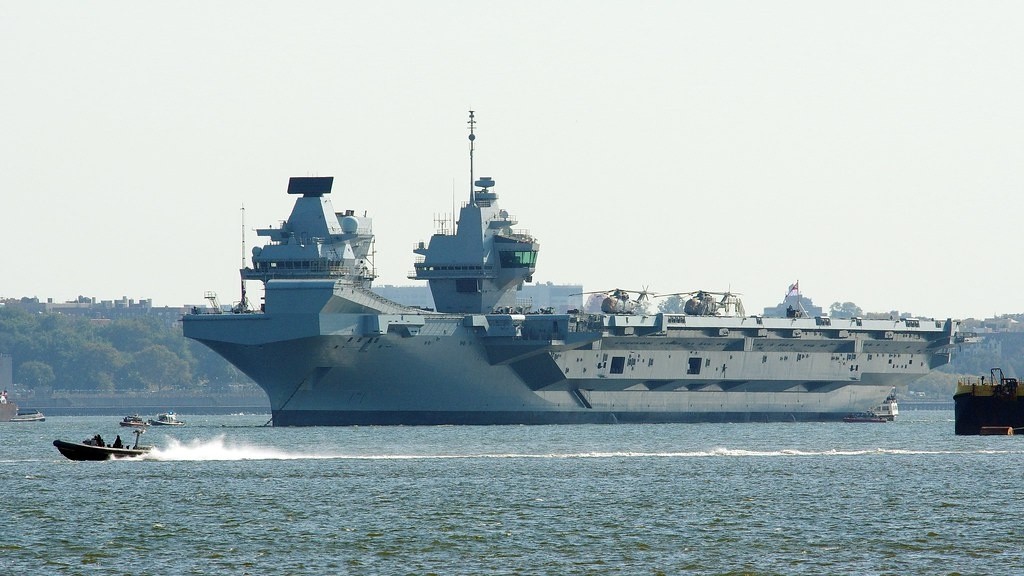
[792,282,799,290]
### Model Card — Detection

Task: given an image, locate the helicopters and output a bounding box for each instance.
[568,284,659,316]
[653,285,745,317]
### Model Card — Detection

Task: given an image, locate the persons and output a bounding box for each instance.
[94,434,104,446]
[114,435,123,448]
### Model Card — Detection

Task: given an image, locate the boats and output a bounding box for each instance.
[952,367,1024,436]
[866,392,900,421]
[842,411,888,423]
[175,107,968,429]
[119,413,151,427]
[147,412,186,426]
[52,426,158,462]
[10,408,46,422]
[0,401,18,422]
